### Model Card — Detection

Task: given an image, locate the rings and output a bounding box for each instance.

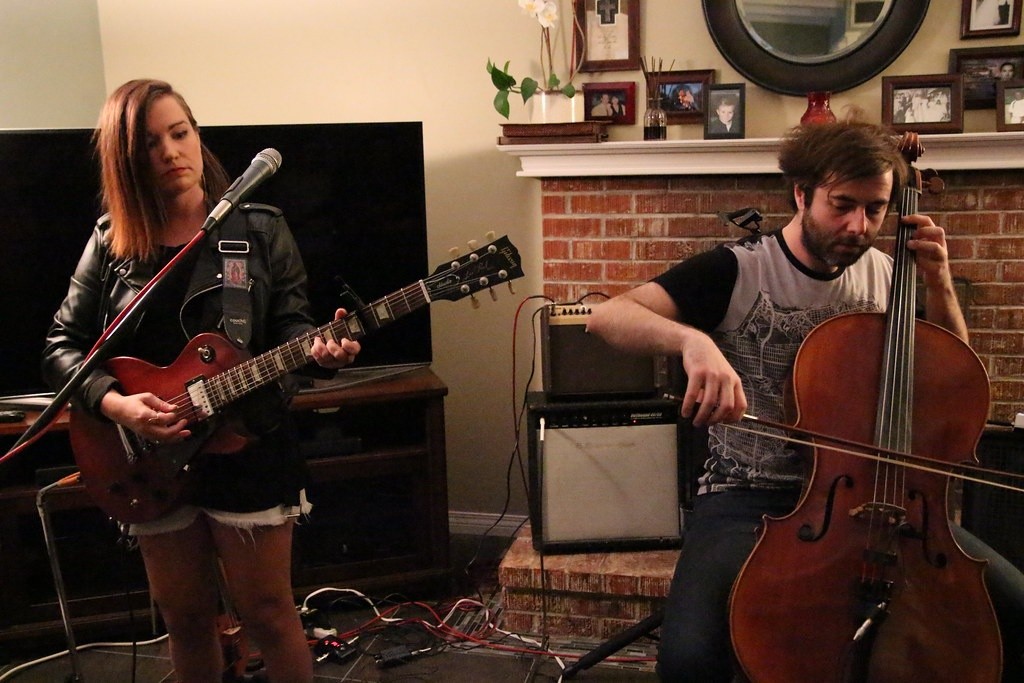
[149,413,160,426]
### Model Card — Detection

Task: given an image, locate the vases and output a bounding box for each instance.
[530,87,582,123]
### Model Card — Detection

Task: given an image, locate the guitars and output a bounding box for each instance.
[68,227,527,527]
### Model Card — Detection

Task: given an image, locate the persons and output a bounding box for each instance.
[992,62,1017,98]
[43,79,361,683]
[671,89,695,110]
[975,0,1010,25]
[588,120,1024,683]
[912,90,947,122]
[1008,90,1024,123]
[591,92,625,117]
[711,93,739,134]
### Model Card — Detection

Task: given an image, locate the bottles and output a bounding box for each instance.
[800,91,836,128]
[644,97,667,141]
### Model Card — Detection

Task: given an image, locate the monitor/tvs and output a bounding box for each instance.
[0,119,433,408]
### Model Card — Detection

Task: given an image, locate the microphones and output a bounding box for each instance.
[200,147,282,235]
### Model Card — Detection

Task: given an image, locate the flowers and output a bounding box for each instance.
[486,0,588,120]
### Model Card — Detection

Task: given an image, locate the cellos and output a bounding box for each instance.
[726,129,1007,683]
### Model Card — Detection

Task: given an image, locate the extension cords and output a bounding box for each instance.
[303,626,338,639]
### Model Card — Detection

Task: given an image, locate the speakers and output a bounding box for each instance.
[956,422,1024,576]
[525,388,692,551]
[539,304,671,396]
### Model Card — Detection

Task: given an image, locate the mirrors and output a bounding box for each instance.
[702,0,931,97]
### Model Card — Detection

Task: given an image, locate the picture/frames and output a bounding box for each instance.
[879,73,965,131]
[645,67,717,125]
[575,0,640,73]
[950,44,1024,110]
[582,80,637,125]
[995,78,1024,131]
[703,82,746,139]
[958,0,1021,40]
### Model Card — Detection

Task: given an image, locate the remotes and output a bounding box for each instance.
[0,410,25,424]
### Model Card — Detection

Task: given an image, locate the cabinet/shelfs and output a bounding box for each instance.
[0,357,465,658]
